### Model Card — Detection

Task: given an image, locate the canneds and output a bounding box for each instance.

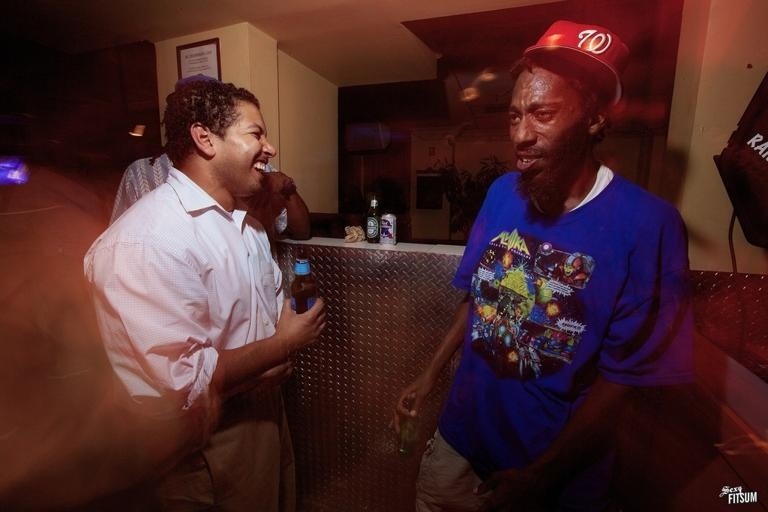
[380,214,396,246]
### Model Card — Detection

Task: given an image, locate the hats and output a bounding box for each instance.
[522,20,631,107]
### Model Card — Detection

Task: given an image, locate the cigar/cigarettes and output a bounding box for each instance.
[399,402,410,454]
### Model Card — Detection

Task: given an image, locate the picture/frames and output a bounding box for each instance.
[176,38,223,82]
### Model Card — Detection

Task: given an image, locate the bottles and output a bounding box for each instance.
[288,244,316,313]
[395,391,420,463]
[366,190,379,244]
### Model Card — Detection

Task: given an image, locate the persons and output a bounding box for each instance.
[106,70,317,242]
[371,18,699,512]
[80,81,331,511]
